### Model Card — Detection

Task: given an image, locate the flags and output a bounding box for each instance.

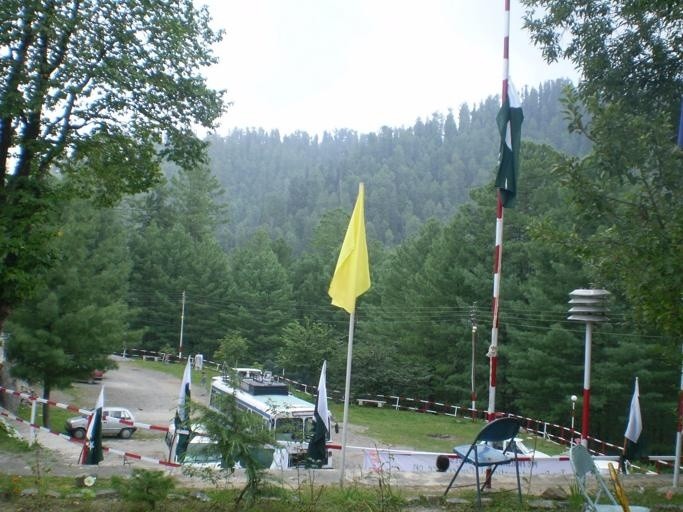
[327,180,372,316]
[82,386,105,466]
[493,69,526,210]
[621,374,643,475]
[165,356,193,465]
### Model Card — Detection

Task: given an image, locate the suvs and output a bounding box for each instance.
[65,407,138,439]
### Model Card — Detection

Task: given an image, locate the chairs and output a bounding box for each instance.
[441,417,523,505]
[569,443,650,512]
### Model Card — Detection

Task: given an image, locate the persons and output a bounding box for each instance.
[302,359,329,469]
[200,371,207,396]
[192,350,203,372]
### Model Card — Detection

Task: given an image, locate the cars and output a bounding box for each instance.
[72,364,104,384]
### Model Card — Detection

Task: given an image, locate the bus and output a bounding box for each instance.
[207,367,338,468]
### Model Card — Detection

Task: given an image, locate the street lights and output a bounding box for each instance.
[469,302,476,410]
[570,395,578,447]
